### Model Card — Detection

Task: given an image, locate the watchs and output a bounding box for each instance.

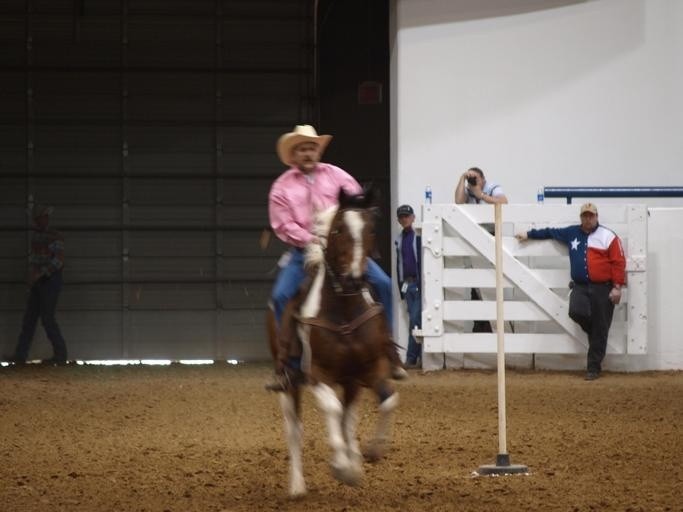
[612,284,621,290]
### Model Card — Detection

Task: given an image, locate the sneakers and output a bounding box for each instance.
[263,371,304,392]
[584,370,600,380]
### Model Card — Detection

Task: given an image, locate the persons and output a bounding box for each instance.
[514,202,627,381]
[454,166,514,336]
[263,123,409,392]
[8,201,66,371]
[393,202,423,371]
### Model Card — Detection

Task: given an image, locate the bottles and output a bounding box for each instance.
[424,183,432,205]
[537,185,544,205]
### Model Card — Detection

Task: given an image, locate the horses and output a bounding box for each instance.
[266,181,401,502]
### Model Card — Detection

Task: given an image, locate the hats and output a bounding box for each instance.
[275,123,335,169]
[580,203,598,218]
[396,205,413,217]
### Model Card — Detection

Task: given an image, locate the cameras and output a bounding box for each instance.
[468,176,477,185]
